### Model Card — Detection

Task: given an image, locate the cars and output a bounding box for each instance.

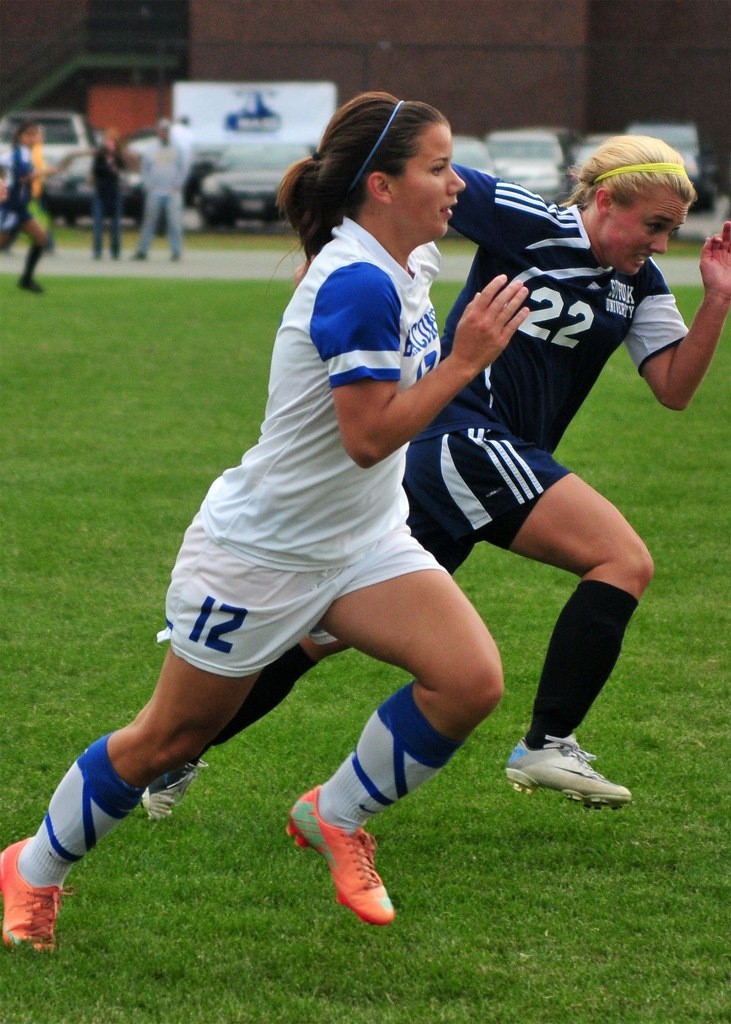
[451,143,493,190]
[199,140,326,233]
[38,131,164,225]
[570,134,616,184]
[490,130,566,202]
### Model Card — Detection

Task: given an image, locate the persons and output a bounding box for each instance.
[130,118,187,262]
[0,91,530,952]
[140,136,731,817]
[89,128,130,261]
[0,121,64,293]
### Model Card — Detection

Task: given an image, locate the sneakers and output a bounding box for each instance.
[141,759,209,820]
[506,733,632,810]
[285,786,395,926]
[0,837,76,951]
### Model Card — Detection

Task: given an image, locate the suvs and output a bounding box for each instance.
[0,112,92,192]
[630,122,716,212]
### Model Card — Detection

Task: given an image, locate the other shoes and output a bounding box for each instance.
[18,277,42,295]
[129,251,146,259]
